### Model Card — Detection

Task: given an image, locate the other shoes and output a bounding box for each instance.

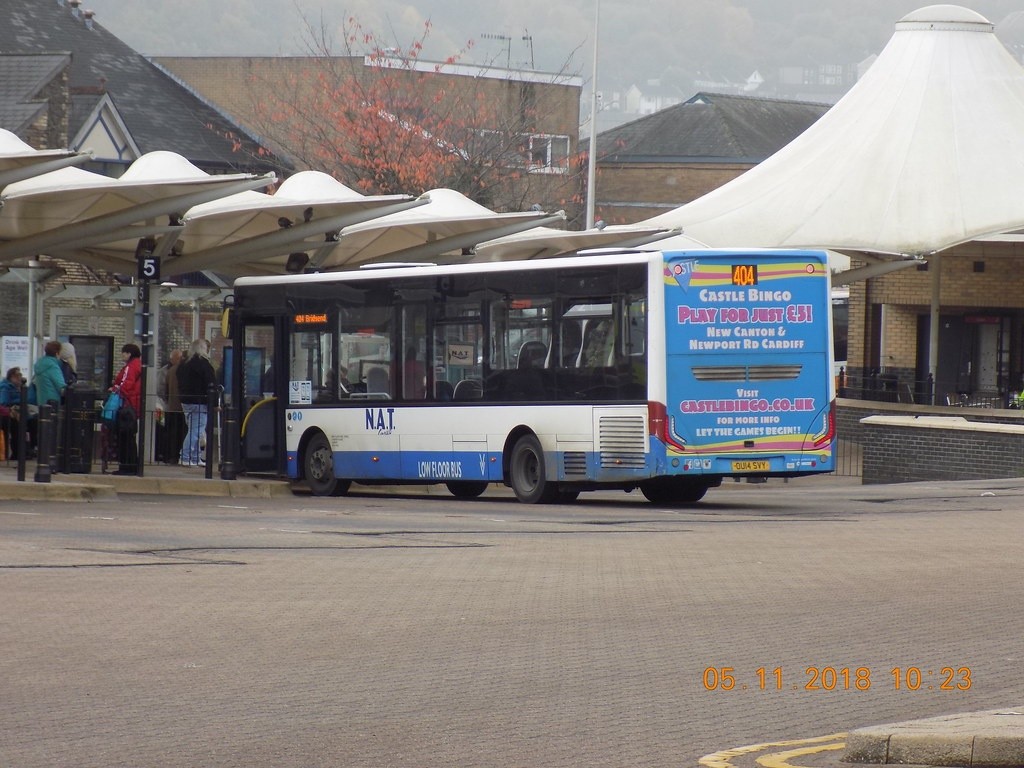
[112,468,137,476]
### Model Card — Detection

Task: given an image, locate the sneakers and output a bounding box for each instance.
[190,459,206,466]
[178,459,193,466]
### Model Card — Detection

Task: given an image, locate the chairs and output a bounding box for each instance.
[452,379,483,399]
[607,292,647,367]
[941,392,962,407]
[544,317,583,369]
[423,380,455,400]
[367,367,390,399]
[576,318,615,368]
[960,393,994,408]
[516,340,547,369]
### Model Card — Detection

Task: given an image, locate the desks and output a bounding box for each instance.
[975,392,1005,409]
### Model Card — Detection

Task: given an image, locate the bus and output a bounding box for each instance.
[219,247,839,506]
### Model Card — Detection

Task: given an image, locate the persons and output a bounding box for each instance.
[324,346,428,401]
[0,338,219,477]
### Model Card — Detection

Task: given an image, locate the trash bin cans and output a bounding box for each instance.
[876,373,899,401]
[58,385,93,472]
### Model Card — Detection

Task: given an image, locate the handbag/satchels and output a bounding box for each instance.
[100,390,124,421]
[60,358,78,384]
